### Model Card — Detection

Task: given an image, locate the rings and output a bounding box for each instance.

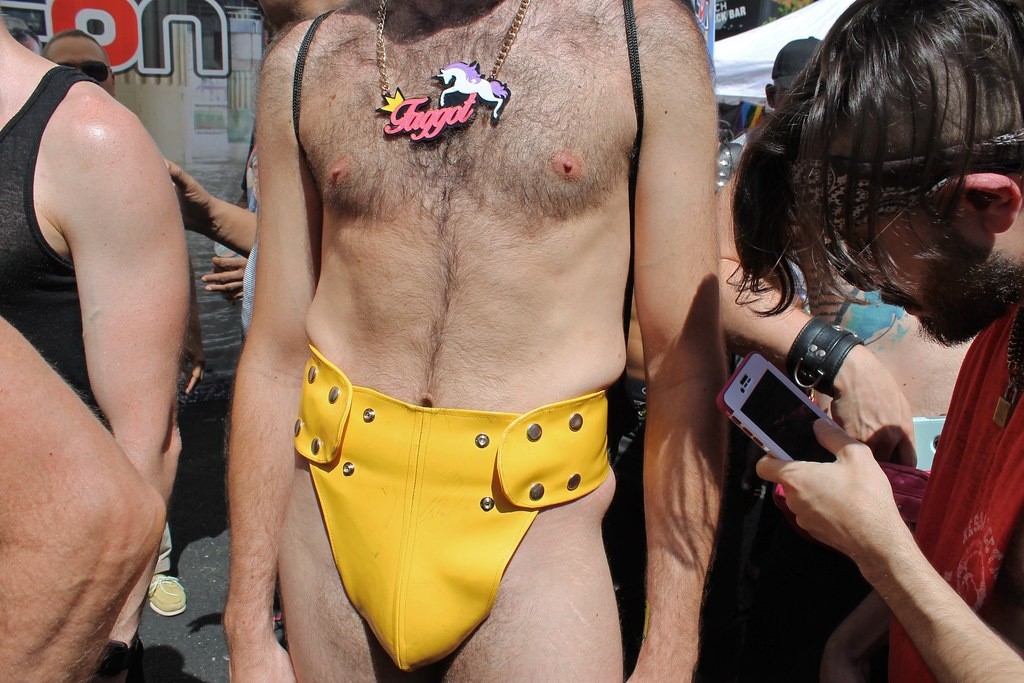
[182,345,193,361]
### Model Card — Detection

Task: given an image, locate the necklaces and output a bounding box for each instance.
[375,0,530,140]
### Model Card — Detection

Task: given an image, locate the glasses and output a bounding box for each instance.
[809,176,949,307]
[58,61,109,82]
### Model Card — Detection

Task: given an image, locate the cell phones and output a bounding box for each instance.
[715,351,842,463]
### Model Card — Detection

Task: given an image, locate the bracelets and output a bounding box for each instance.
[783,312,865,394]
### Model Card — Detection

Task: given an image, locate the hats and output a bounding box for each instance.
[772,36,822,89]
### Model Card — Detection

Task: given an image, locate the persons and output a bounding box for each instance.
[0,0,1024,683]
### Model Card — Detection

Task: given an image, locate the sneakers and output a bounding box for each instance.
[148,573,186,616]
